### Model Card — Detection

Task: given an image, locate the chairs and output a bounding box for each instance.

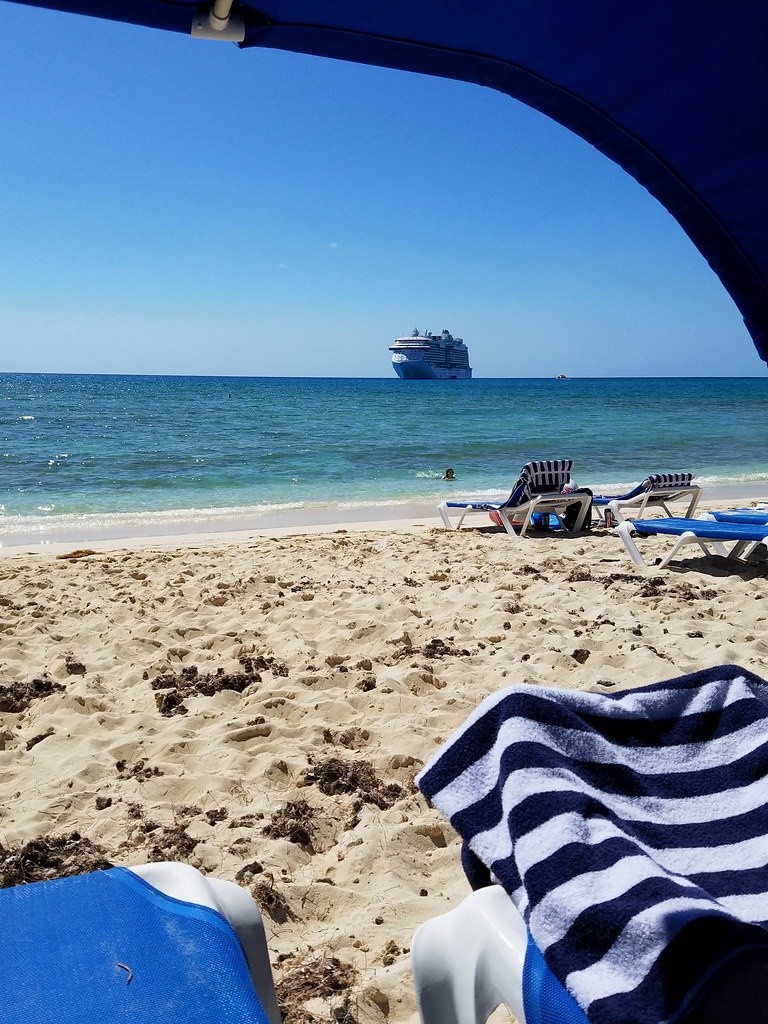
[592,472,702,525]
[615,510,768,578]
[437,459,593,538]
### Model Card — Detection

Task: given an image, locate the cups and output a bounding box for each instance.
[542,515,549,528]
[605,509,611,528]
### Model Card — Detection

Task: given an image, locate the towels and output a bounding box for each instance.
[412,666,767,1023]
[477,458,574,512]
[593,472,693,499]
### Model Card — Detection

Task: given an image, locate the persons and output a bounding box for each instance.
[443,468,455,479]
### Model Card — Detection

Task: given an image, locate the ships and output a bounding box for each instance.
[388,329,473,379]
